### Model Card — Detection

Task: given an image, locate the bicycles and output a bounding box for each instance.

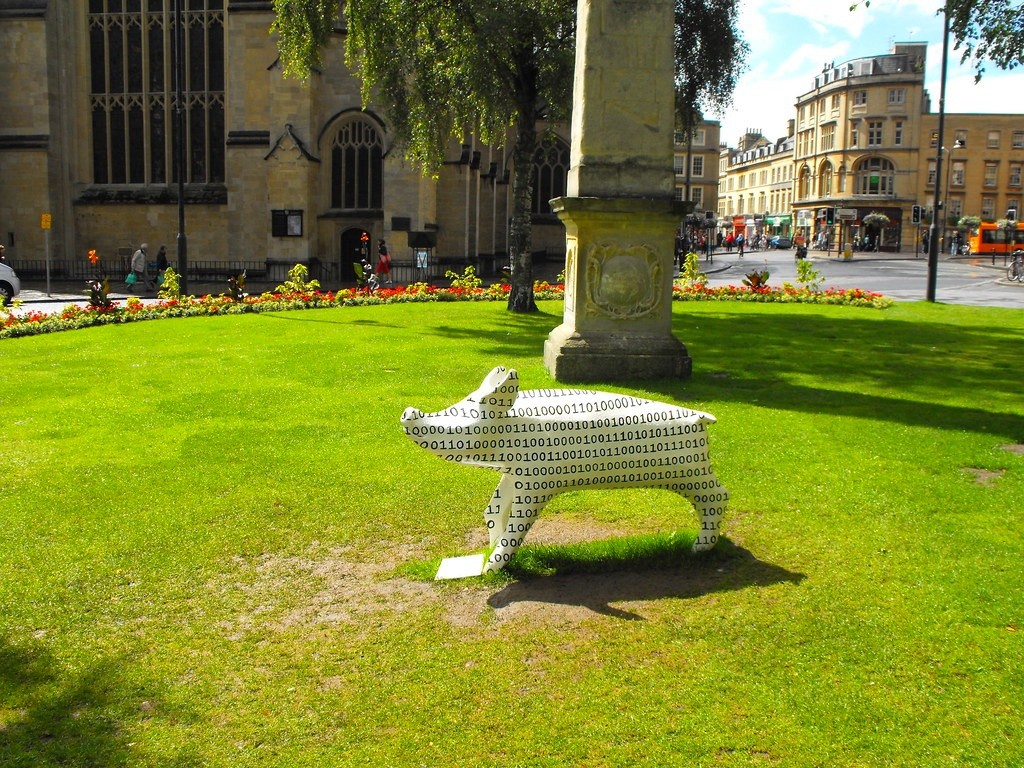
[1006,252,1024,282]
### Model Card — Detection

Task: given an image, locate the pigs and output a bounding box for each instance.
[402,366,729,581]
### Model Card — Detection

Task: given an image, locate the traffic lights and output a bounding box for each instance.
[912,205,926,225]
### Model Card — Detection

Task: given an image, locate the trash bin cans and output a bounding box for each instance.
[679,251,690,272]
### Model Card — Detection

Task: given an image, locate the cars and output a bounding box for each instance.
[771,235,791,249]
[0,263,20,306]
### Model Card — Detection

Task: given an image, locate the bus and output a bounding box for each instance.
[968,221,1024,254]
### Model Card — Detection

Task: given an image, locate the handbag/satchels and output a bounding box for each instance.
[157,272,165,285]
[379,251,391,263]
[124,272,137,285]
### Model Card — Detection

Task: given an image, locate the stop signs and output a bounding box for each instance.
[827,208,835,226]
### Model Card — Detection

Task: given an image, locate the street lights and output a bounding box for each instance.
[942,140,961,253]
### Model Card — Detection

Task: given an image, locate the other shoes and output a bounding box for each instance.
[384,281,392,283]
[127,287,135,292]
[146,288,154,292]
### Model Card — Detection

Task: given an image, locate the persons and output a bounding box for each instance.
[0,245,6,262]
[851,233,880,253]
[674,227,830,265]
[950,231,969,256]
[921,229,928,254]
[125,243,156,292]
[149,245,169,289]
[374,239,393,284]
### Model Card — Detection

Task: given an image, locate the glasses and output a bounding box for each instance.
[165,247,167,249]
